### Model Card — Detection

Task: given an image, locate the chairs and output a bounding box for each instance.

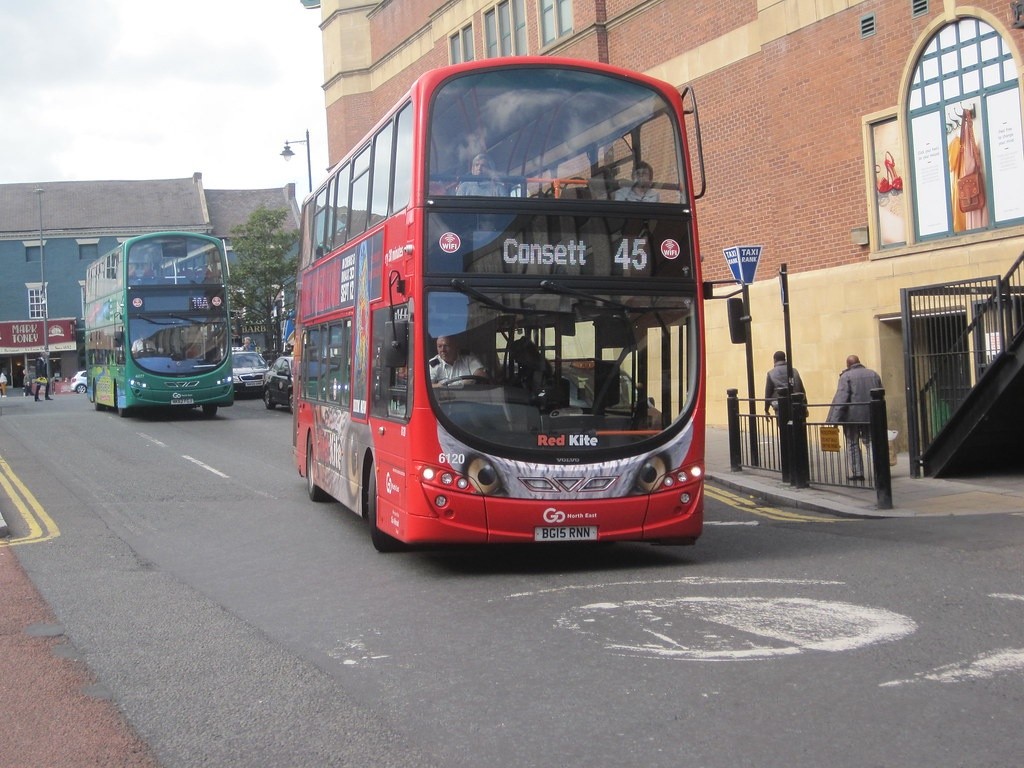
[172,265,209,281]
[528,174,623,201]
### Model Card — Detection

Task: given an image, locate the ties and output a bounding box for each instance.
[143,340,147,352]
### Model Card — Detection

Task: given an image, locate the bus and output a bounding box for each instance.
[85,230,237,418]
[293,55,753,554]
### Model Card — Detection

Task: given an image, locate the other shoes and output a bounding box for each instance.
[2,395,7,397]
[36,399,42,401]
[45,397,53,400]
[849,476,864,481]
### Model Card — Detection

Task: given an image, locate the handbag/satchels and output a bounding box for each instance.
[888,429,899,465]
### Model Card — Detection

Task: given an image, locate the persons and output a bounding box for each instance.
[132,333,157,355]
[0,373,8,397]
[509,336,554,389]
[612,161,661,203]
[824,354,884,480]
[764,350,809,427]
[22,369,35,396]
[456,153,510,197]
[428,334,492,387]
[34,351,53,402]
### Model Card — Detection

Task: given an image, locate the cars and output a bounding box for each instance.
[230,346,273,399]
[70,370,87,394]
[262,356,293,413]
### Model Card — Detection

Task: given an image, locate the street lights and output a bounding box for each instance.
[33,188,52,382]
[281,128,313,194]
